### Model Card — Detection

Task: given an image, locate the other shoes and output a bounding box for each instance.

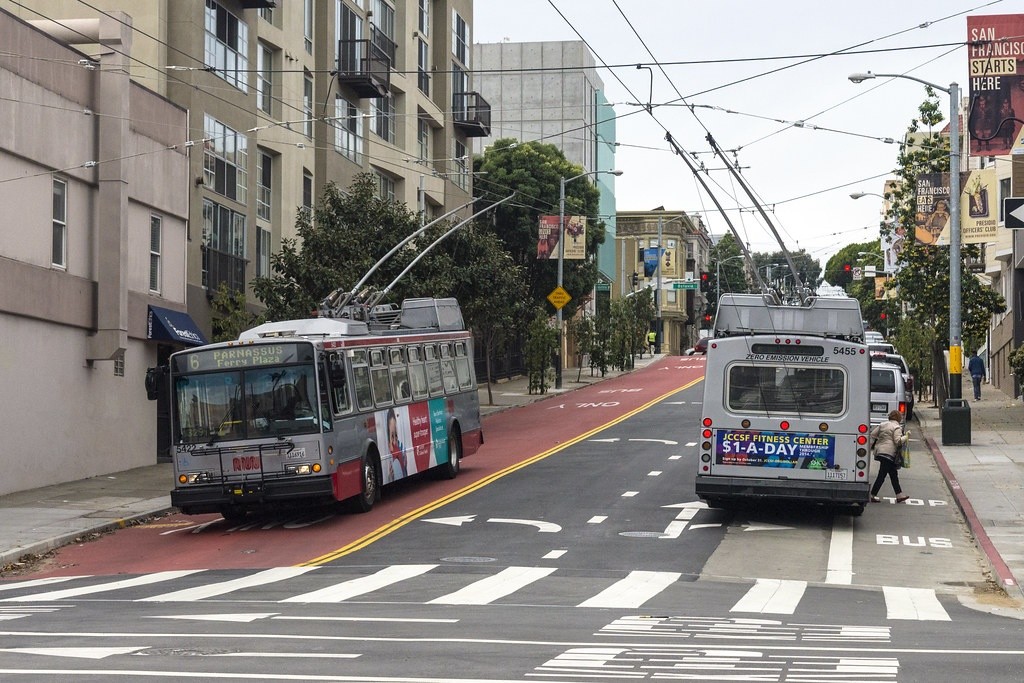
[978,397,981,399]
[871,497,881,503]
[974,399,979,401]
[897,496,909,503]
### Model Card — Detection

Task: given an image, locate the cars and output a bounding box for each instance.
[863,321,899,354]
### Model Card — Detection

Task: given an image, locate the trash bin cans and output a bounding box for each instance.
[940,398,971,446]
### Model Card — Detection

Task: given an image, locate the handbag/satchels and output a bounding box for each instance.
[894,432,910,469]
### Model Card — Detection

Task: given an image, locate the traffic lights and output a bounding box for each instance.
[700,272,709,281]
[706,316,710,320]
[845,265,851,271]
[880,313,887,319]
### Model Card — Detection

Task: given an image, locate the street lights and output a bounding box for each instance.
[717,256,745,307]
[857,258,867,262]
[555,169,623,388]
[654,215,702,354]
[784,273,800,292]
[858,252,889,336]
[758,264,778,287]
[850,192,893,203]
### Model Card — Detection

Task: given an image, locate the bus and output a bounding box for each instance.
[694,293,869,517]
[849,71,971,446]
[145,298,485,523]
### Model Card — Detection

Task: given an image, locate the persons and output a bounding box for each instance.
[386,408,408,483]
[870,410,912,503]
[968,350,987,401]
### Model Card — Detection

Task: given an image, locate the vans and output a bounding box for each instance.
[867,363,907,447]
[868,354,914,420]
